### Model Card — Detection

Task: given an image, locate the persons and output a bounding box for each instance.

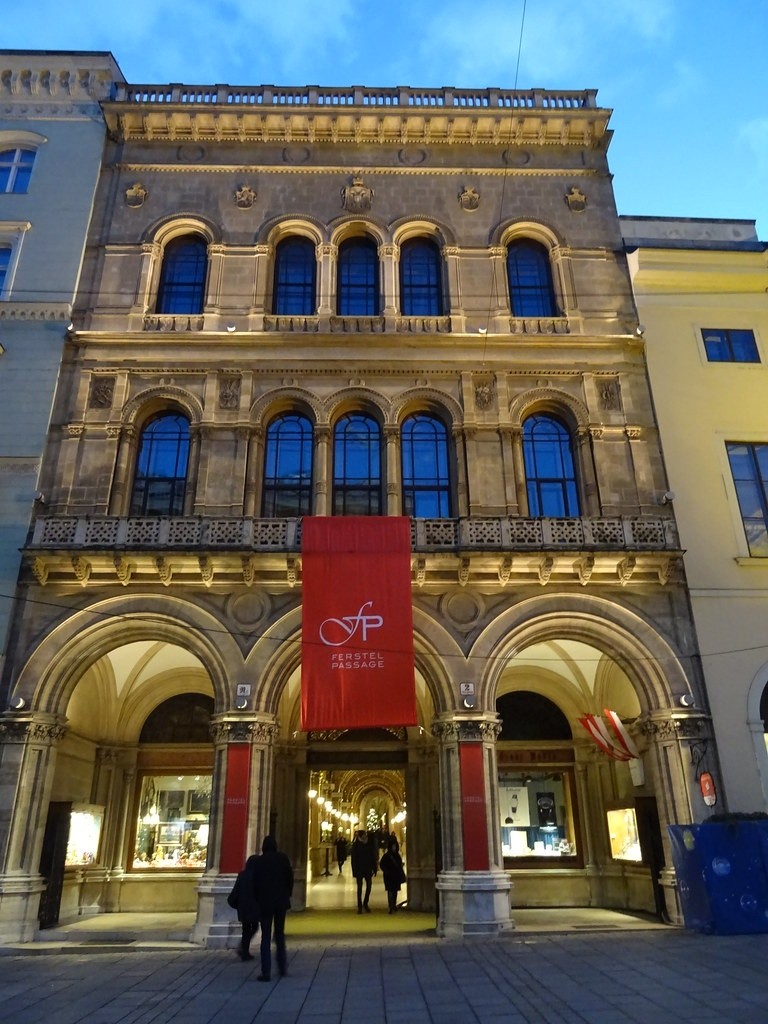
[254,835,295,982]
[237,855,264,961]
[335,828,405,914]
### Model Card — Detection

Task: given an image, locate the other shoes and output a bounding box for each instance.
[236,946,255,962]
[258,969,288,981]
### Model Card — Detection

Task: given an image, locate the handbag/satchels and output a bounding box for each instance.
[401,870,406,883]
